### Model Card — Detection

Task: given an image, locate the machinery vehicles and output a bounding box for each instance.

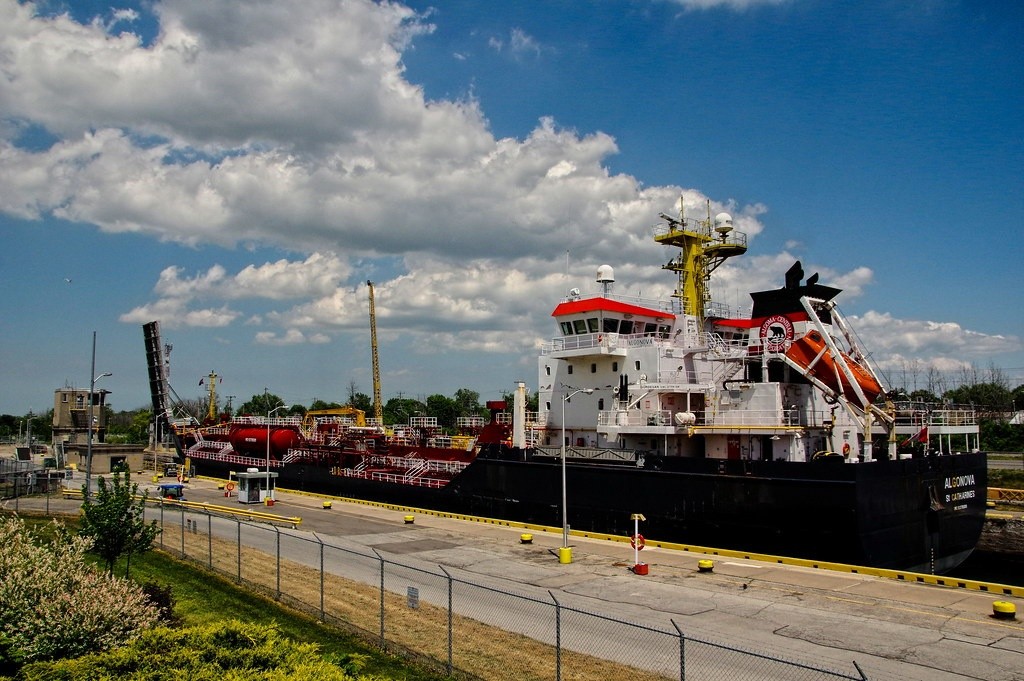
[157,485,188,509]
[176,464,190,483]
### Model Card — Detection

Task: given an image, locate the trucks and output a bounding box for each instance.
[163,463,177,477]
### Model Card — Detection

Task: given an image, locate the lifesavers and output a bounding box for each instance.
[631,534,645,551]
[178,475,185,482]
[843,443,850,456]
[598,335,602,342]
[226,482,235,491]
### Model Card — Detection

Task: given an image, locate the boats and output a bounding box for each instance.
[169,197,988,579]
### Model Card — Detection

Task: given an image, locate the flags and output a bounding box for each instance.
[902,427,927,446]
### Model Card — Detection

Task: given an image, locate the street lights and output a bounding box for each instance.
[267,406,290,499]
[898,393,913,448]
[27,416,39,451]
[561,389,593,549]
[398,409,409,427]
[155,410,171,477]
[86,372,113,503]
[20,421,22,446]
